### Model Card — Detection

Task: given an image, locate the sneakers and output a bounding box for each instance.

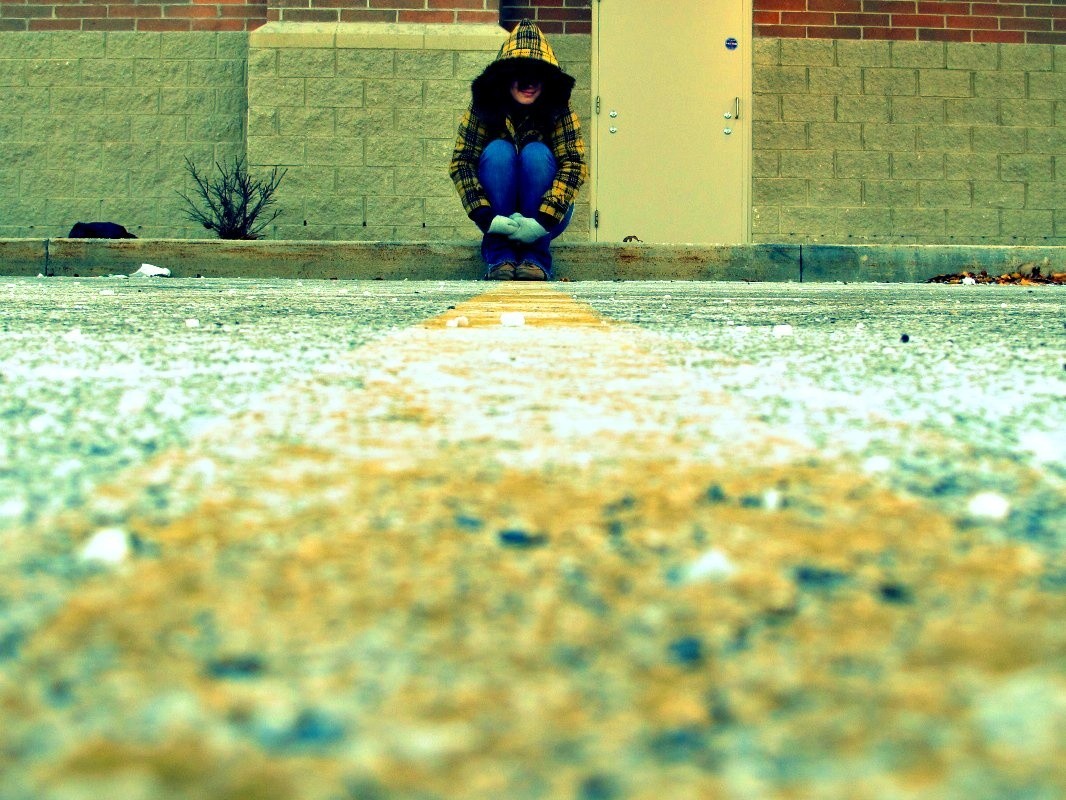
[516,261,545,280]
[488,262,516,281]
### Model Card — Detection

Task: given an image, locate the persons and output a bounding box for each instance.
[450,18,587,281]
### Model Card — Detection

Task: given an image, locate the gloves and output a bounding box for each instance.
[509,215,548,242]
[488,212,522,235]
